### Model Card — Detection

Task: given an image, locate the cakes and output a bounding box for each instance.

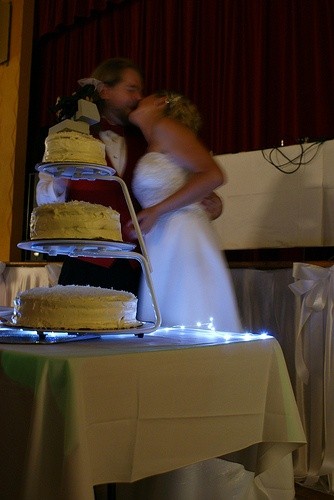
[40,129,107,166]
[29,199,123,242]
[12,285,139,329]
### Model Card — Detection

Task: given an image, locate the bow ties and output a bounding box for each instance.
[100,118,125,138]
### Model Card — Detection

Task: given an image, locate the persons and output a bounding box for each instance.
[35,56,223,319]
[129,88,246,500]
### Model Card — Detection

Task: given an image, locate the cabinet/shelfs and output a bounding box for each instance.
[3,161,162,344]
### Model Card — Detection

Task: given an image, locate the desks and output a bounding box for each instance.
[0,327,307,499]
